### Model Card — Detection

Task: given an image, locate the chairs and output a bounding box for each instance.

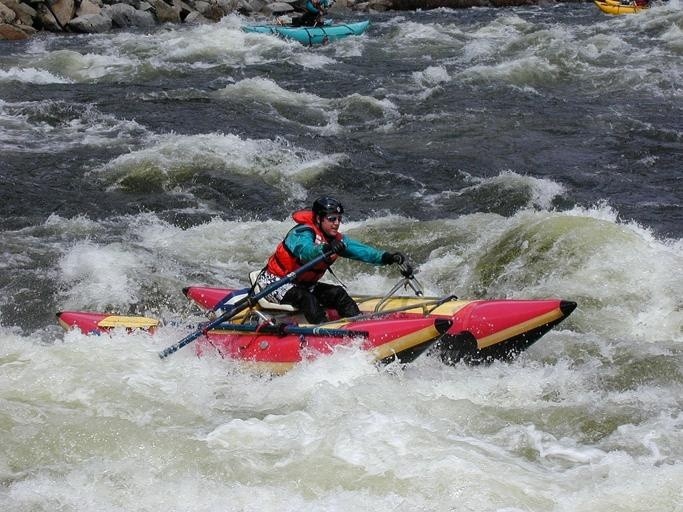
[248,268,298,314]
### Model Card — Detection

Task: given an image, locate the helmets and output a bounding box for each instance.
[313,197,344,216]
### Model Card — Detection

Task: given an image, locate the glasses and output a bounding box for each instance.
[327,215,342,221]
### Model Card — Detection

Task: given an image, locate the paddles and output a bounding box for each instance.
[98,316,368,338]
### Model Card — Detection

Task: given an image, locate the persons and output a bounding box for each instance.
[621,0,650,7]
[256,197,405,325]
[302,0,333,27]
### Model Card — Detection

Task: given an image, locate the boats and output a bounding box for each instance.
[242,20,369,47]
[594,0,652,16]
[56,286,577,377]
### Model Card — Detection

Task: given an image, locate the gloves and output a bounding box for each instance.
[323,240,346,253]
[382,252,404,264]
